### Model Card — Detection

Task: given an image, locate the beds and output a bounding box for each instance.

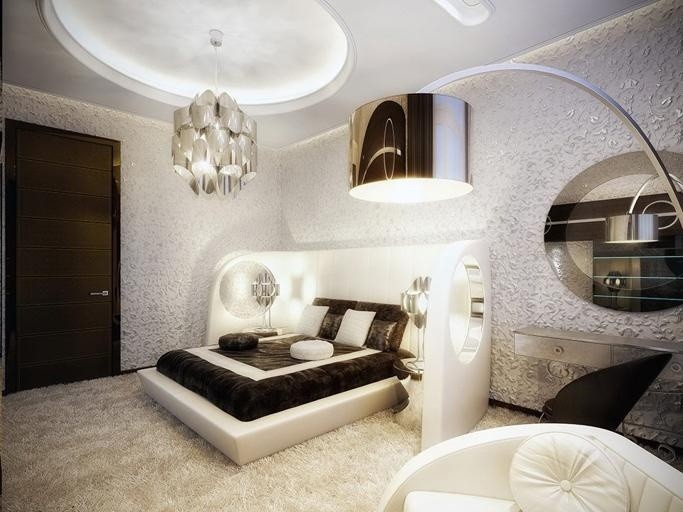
[138,298,417,468]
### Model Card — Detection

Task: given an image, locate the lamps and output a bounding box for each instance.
[603,171,683,245]
[346,61,683,231]
[401,275,433,368]
[251,268,280,331]
[171,30,258,201]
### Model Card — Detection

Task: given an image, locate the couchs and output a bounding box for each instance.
[377,421,683,512]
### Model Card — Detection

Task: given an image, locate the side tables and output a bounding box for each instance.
[393,358,423,417]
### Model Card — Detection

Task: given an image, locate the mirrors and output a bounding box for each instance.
[541,148,683,312]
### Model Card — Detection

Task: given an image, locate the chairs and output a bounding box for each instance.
[536,352,673,434]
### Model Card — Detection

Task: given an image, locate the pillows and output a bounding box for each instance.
[365,319,398,352]
[508,429,631,512]
[218,332,259,351]
[402,490,519,512]
[289,339,334,361]
[333,309,376,349]
[316,311,344,341]
